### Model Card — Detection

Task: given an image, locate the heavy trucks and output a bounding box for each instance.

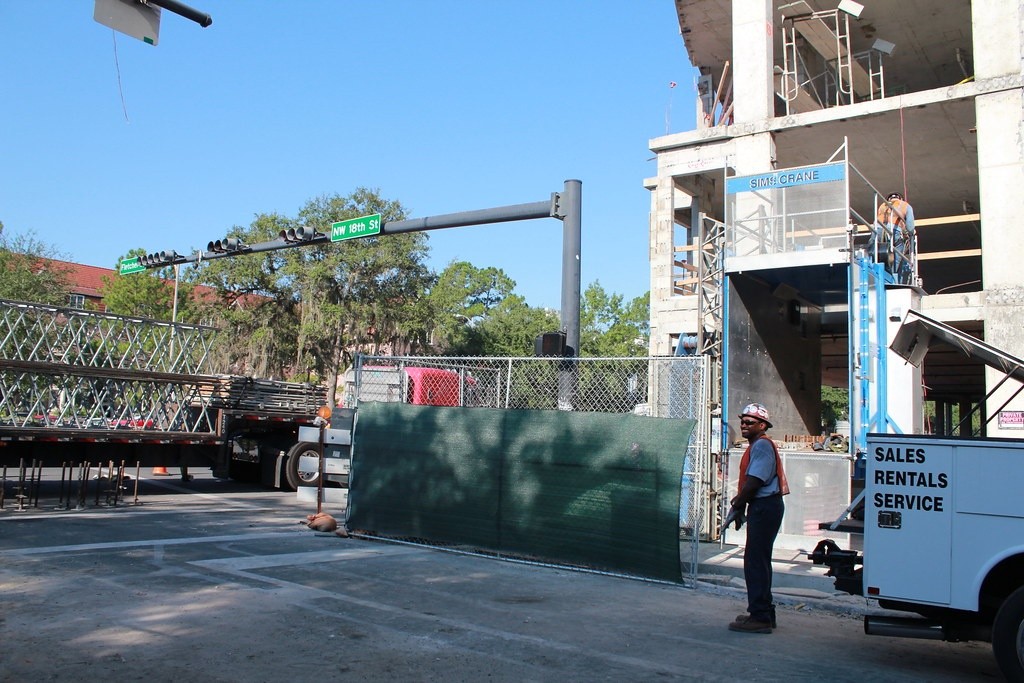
[2,367,480,491]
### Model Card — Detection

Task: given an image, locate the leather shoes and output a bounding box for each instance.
[728,618,772,634]
[736,615,776,628]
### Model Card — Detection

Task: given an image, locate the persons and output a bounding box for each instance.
[868,192,918,276]
[712,403,786,636]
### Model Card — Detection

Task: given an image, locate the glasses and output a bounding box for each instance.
[741,420,761,426]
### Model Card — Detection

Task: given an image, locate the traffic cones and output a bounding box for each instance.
[152,467,171,476]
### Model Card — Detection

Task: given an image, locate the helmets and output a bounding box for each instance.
[742,402,774,428]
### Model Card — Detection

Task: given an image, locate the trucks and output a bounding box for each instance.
[809,433,1024,683]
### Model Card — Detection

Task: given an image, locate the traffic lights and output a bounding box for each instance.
[136,250,175,268]
[535,332,562,356]
[206,237,243,254]
[278,226,314,244]
